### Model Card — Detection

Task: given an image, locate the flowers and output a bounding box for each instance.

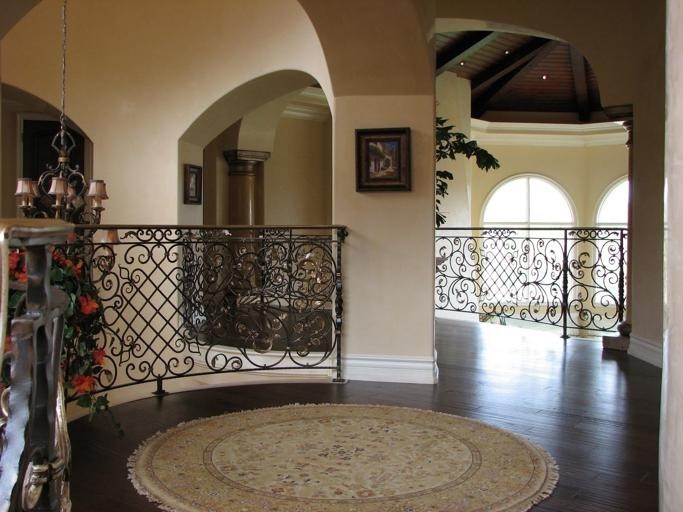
[0,244,122,438]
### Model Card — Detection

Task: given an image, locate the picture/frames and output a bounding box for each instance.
[183,162,203,205]
[353,128,411,192]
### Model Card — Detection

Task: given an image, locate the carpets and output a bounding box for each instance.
[126,402,559,511]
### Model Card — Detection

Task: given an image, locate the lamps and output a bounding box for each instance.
[11,0,120,262]
